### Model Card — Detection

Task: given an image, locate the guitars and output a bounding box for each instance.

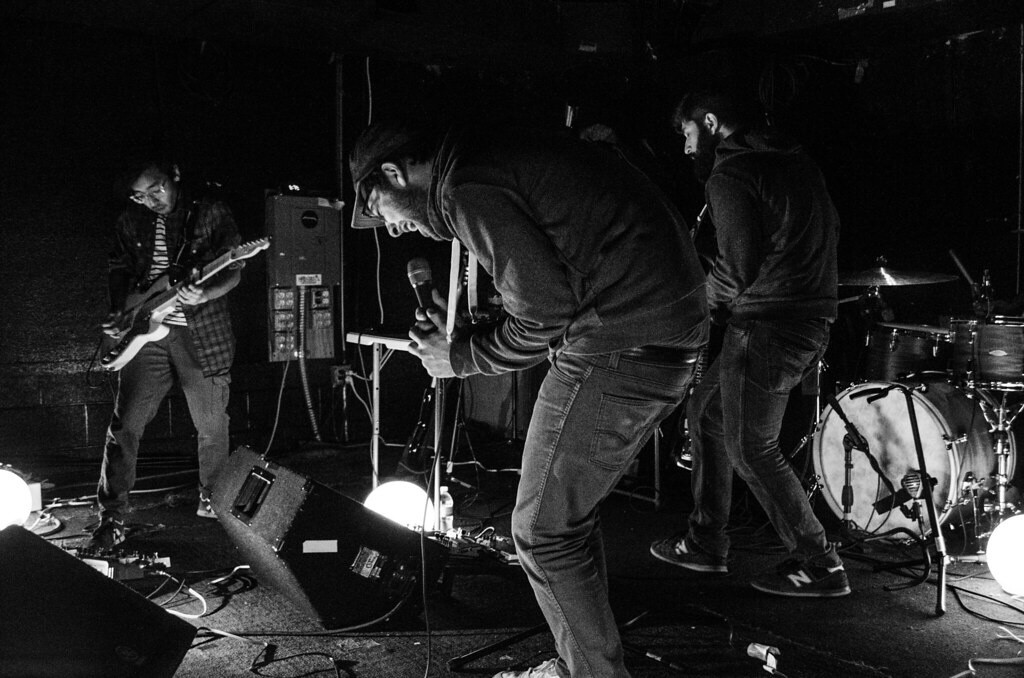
[98,234,274,372]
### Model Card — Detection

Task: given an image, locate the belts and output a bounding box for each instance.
[617,347,704,364]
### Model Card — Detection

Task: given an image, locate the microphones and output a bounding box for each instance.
[408,259,444,334]
[980,270,994,302]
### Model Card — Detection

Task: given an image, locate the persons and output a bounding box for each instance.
[74,141,245,558]
[649,88,852,597]
[350,110,711,678]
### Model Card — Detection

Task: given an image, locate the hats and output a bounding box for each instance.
[350,88,429,229]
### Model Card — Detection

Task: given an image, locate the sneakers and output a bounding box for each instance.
[196,500,215,517]
[748,557,851,596]
[649,539,727,572]
[78,517,127,554]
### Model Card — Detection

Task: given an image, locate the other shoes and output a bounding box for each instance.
[491,657,564,678]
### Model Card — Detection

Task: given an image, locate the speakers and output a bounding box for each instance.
[198,444,451,633]
[0,526,197,678]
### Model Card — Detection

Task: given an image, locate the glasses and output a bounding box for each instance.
[130,175,166,204]
[361,185,385,222]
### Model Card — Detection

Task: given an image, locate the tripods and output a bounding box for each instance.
[849,382,991,615]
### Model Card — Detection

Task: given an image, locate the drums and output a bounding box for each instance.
[866,321,956,385]
[811,381,1018,546]
[949,314,1024,391]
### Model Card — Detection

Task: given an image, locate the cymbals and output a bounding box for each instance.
[836,267,961,288]
